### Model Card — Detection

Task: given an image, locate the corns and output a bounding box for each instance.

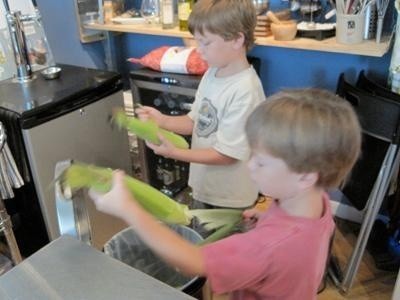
[112,111,188,151]
[63,160,191,226]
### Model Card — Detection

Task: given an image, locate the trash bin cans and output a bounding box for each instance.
[101,224,206,292]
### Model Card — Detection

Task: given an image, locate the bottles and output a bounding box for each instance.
[177,0,191,32]
[161,0,174,28]
[155,156,186,189]
[154,93,193,108]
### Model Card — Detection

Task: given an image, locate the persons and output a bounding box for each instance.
[86,87,363,300]
[133,0,267,238]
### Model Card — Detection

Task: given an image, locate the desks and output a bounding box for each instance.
[0,232,196,300]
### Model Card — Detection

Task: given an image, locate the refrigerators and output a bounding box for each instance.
[0,63,151,257]
